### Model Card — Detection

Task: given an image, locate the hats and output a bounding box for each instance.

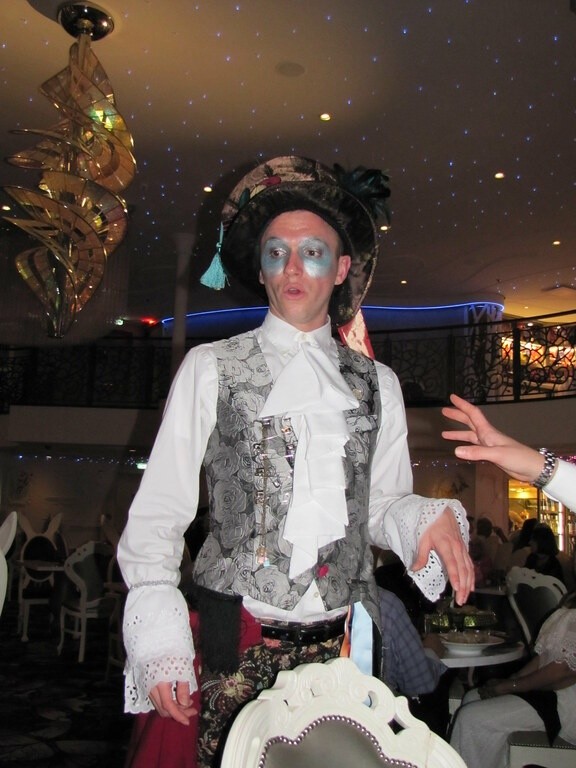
[199,154,392,326]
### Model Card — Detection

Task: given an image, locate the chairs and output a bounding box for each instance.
[0,511,128,674]
[507,566,567,644]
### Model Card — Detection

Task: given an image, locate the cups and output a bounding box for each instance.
[423,614,435,636]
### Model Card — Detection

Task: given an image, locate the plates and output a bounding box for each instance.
[436,632,506,654]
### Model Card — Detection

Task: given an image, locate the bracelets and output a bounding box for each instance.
[513,679,523,691]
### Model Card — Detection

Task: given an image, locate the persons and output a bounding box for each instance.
[365,393,575,757]
[118,156,474,768]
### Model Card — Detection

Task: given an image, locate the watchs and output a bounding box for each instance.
[531,447,558,489]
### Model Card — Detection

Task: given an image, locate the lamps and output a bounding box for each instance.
[1,1,139,338]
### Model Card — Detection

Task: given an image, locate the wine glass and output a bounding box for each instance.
[435,597,455,637]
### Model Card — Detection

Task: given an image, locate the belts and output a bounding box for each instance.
[262,613,345,645]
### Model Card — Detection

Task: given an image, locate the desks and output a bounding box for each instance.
[473,586,507,608]
[220,657,576,768]
[14,560,64,573]
[434,640,526,686]
